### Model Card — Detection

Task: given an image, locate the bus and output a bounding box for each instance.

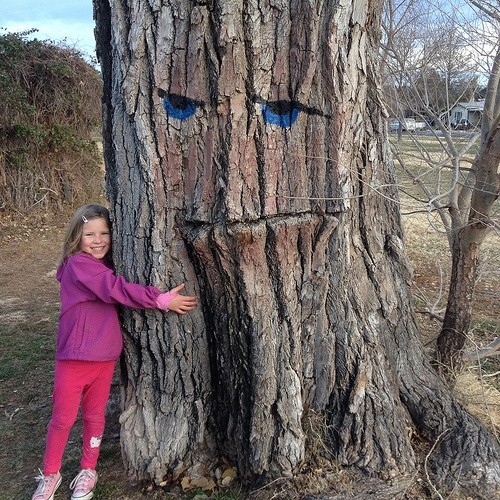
[389,118,415,132]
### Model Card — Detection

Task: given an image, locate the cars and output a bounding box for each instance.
[454,119,470,130]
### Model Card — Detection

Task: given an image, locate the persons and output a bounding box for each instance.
[29,202,199,500]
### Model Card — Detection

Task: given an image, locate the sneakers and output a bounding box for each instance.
[71,470,98,500]
[32,470,62,500]
